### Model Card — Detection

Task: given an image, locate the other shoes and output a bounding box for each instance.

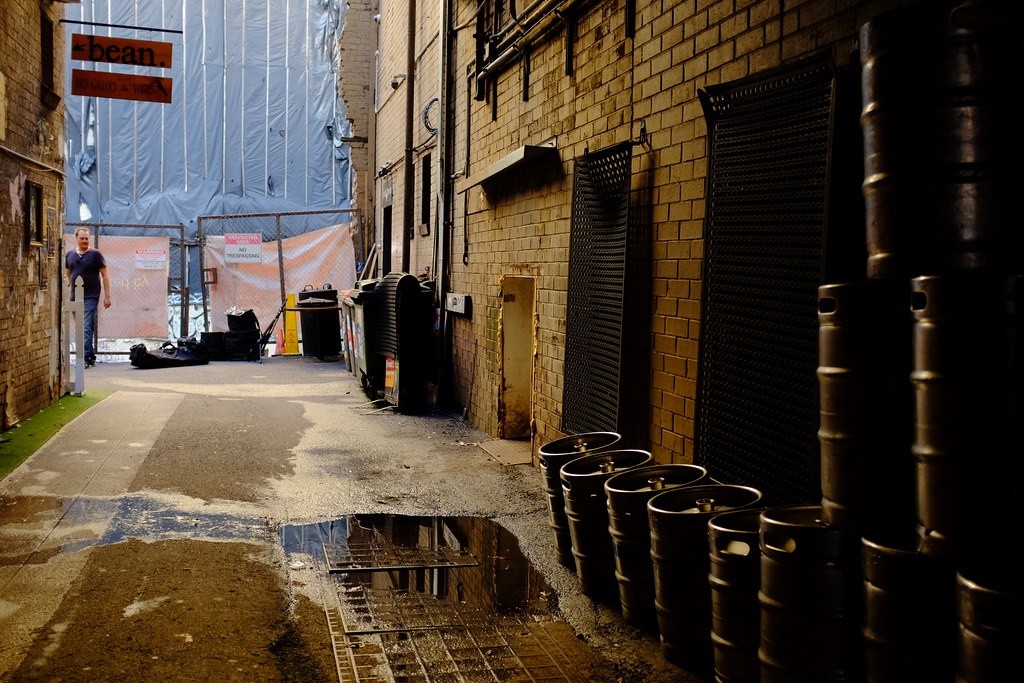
[85,354,96,368]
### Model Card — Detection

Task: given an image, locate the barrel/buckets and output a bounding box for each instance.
[536,11,1024,683]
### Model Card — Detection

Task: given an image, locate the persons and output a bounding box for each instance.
[65,227,111,370]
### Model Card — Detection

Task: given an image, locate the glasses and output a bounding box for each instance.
[77,254,83,263]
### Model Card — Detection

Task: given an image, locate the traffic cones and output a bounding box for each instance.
[271,328,285,357]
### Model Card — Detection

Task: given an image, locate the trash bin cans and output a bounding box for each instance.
[298,284,342,355]
[338,277,437,404]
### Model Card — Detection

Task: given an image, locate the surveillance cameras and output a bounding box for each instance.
[374,14,380,24]
